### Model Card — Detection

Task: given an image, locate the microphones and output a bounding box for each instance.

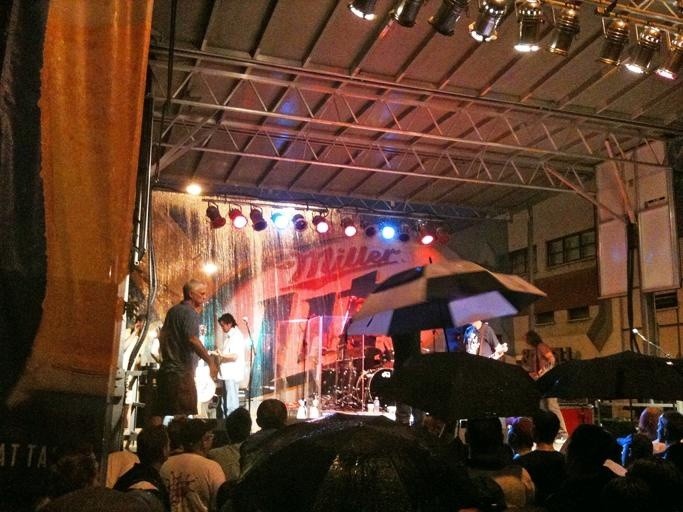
[242,316,249,324]
[631,327,646,341]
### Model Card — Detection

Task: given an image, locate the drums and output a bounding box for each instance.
[356,367,399,411]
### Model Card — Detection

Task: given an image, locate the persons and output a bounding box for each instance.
[112,426,171,512]
[638,407,667,451]
[526,330,556,379]
[213,313,245,420]
[206,408,258,480]
[150,279,218,427]
[462,313,495,360]
[240,400,302,479]
[160,419,227,512]
[457,416,535,512]
[603,434,674,495]
[652,411,683,471]
[511,411,580,511]
[43,427,99,489]
[504,418,535,458]
[625,458,682,501]
[606,476,654,511]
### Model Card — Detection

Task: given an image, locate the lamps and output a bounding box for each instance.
[206,206,226,229]
[339,216,356,237]
[389,0,424,28]
[654,28,683,80]
[413,221,433,245]
[427,0,471,37]
[346,0,378,21]
[468,0,508,41]
[250,210,267,231]
[598,18,630,65]
[292,214,308,232]
[229,208,248,228]
[624,23,659,74]
[547,2,581,53]
[433,223,449,244]
[398,221,412,242]
[359,219,376,238]
[514,1,544,50]
[312,215,330,233]
[377,219,395,240]
[271,212,288,229]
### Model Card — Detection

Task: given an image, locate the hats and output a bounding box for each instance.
[179,417,217,444]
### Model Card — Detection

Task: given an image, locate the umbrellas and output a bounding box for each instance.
[342,260,548,352]
